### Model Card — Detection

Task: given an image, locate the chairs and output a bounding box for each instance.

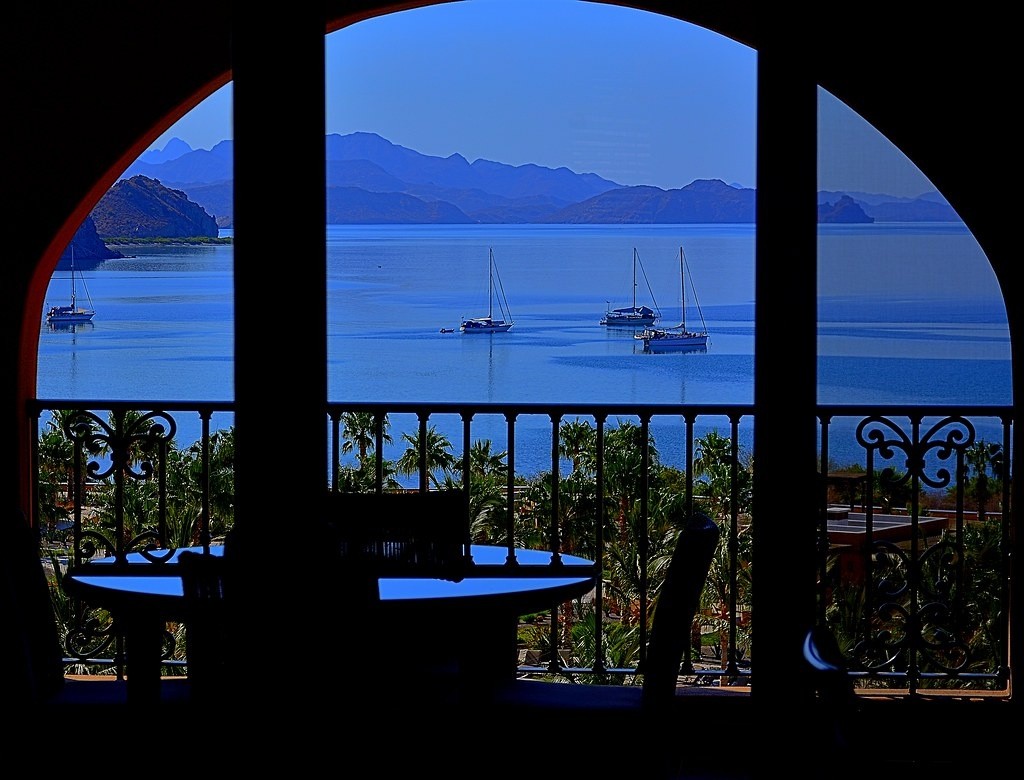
[178,549,379,768]
[314,486,473,572]
[516,513,718,776]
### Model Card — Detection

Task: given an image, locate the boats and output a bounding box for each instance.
[599,247,664,326]
[633,246,710,354]
[460,246,514,332]
[47,243,96,322]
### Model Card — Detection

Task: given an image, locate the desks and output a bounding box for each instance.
[67,573,595,760]
